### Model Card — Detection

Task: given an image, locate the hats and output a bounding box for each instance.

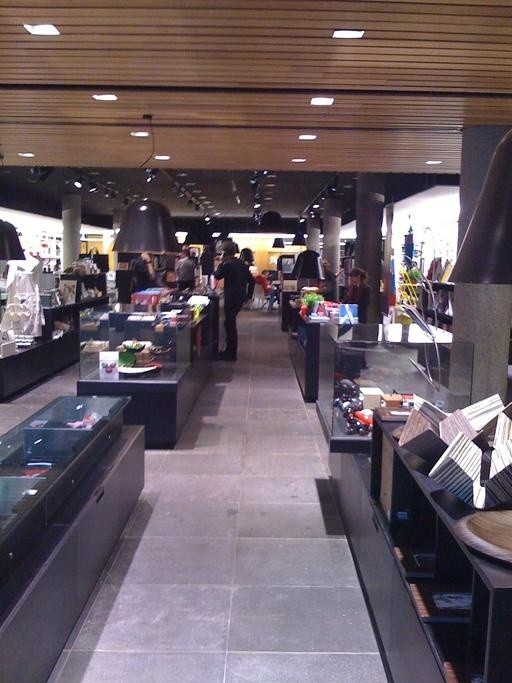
[344,269,363,277]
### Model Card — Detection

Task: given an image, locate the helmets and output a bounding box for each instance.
[221,241,239,253]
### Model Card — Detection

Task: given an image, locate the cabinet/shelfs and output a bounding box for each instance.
[0,270,110,401]
[0,393,147,681]
[77,287,220,449]
[315,321,512,681]
[415,278,456,373]
[277,254,362,402]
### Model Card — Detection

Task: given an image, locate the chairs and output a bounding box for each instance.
[251,282,270,311]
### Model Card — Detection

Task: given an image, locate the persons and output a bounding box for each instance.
[211,240,257,360]
[127,242,374,338]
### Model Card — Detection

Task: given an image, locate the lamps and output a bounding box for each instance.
[290,250,328,292]
[168,171,213,226]
[248,170,268,227]
[69,166,148,211]
[293,172,341,223]
[113,113,180,255]
[272,229,309,250]
[1,154,26,259]
